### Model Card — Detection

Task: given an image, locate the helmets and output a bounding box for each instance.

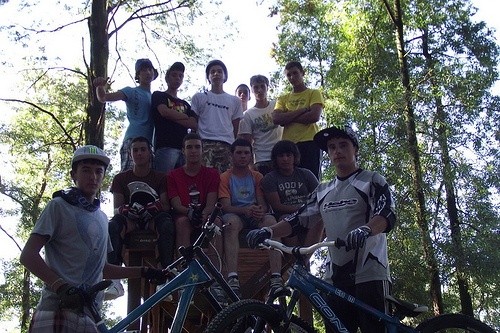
[127,181,160,205]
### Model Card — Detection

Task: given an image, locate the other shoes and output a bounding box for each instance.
[211,283,229,307]
[227,275,240,291]
[269,276,285,293]
[103,280,124,300]
[156,277,174,303]
[177,287,194,305]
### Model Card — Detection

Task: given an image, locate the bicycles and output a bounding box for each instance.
[58,197,320,333]
[204,235,497,333]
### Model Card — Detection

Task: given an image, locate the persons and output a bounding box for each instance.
[236,75,284,176]
[191,60,243,173]
[271,62,325,181]
[19,145,168,333]
[247,125,396,333]
[260,140,325,265]
[92,59,159,172]
[165,132,221,262]
[236,84,251,112]
[152,62,198,171]
[106,136,175,285]
[216,138,285,296]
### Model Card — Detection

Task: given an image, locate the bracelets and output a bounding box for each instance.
[50,278,68,292]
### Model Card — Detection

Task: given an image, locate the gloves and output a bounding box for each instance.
[246,228,269,249]
[140,266,169,284]
[118,204,140,221]
[56,284,83,306]
[345,225,371,252]
[141,201,163,223]
[185,208,203,227]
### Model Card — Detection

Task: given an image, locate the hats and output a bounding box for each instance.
[313,124,359,152]
[72,144,111,172]
[206,60,227,84]
[164,62,185,80]
[135,59,159,83]
